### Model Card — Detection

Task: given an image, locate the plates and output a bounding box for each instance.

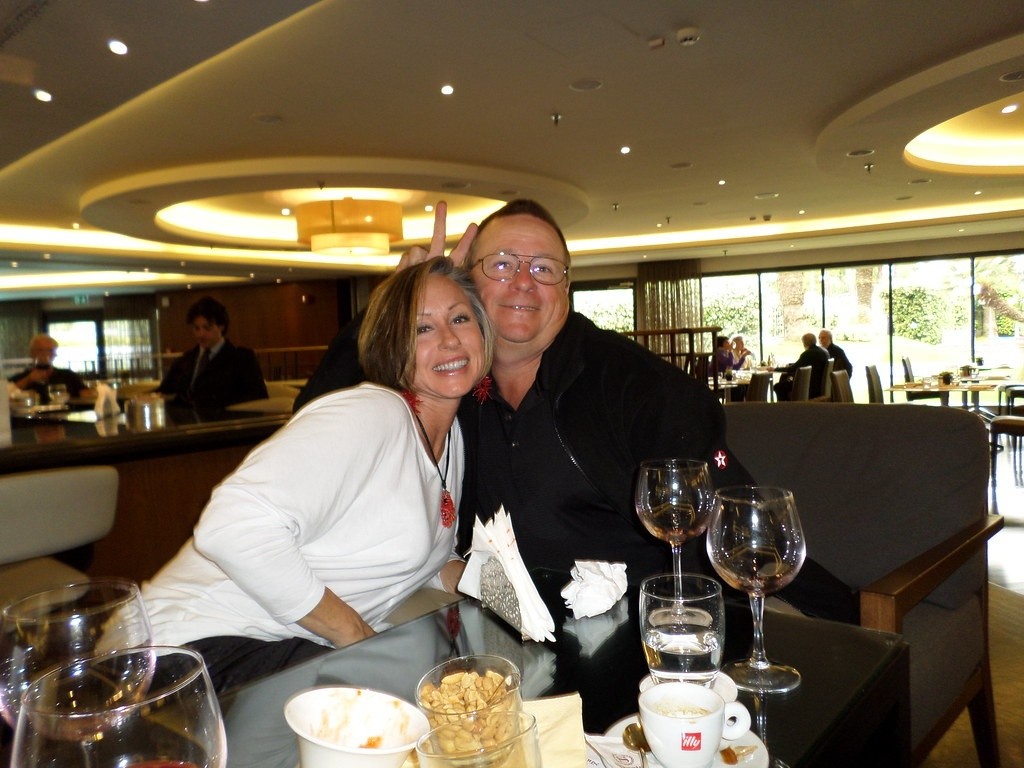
[599,711,770,768]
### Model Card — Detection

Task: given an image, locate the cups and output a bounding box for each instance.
[9,644,229,768]
[49,384,67,404]
[636,681,725,768]
[969,368,979,379]
[416,653,543,768]
[10,390,38,417]
[639,574,724,695]
[283,687,431,768]
[0,579,155,768]
[923,377,932,389]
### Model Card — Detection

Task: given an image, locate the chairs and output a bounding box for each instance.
[0,468,119,628]
[720,359,1024,768]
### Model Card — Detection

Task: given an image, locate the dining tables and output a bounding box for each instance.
[888,365,1012,390]
[218,567,904,768]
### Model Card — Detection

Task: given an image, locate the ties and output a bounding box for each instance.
[195,348,212,376]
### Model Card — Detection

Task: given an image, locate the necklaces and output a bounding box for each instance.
[400,392,456,528]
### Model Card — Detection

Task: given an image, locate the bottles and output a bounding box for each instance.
[938,376,943,387]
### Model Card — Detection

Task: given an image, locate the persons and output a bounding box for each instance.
[731,337,751,370]
[89,256,497,694]
[708,336,751,403]
[8,333,97,403]
[766,334,829,402]
[819,331,852,382]
[291,196,860,625]
[152,298,269,425]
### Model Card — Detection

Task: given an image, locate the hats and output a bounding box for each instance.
[29,333,58,352]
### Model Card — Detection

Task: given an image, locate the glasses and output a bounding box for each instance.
[469,253,569,286]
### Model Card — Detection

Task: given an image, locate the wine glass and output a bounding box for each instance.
[705,485,808,695]
[635,457,712,599]
[738,689,793,768]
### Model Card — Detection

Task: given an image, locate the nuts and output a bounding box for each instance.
[420,669,518,753]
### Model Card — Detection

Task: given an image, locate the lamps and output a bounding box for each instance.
[296,201,401,259]
